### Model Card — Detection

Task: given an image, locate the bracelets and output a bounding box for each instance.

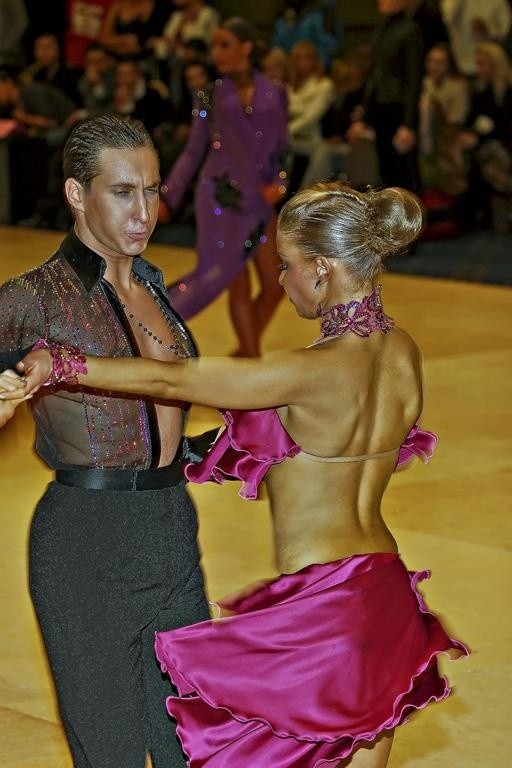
[29,334,92,392]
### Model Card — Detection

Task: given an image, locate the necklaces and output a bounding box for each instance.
[104,267,189,360]
[314,284,396,341]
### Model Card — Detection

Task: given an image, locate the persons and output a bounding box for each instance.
[0,178,474,768]
[0,0,510,288]
[0,112,212,765]
[150,14,296,359]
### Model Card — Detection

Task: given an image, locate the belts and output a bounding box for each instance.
[56,458,184,492]
